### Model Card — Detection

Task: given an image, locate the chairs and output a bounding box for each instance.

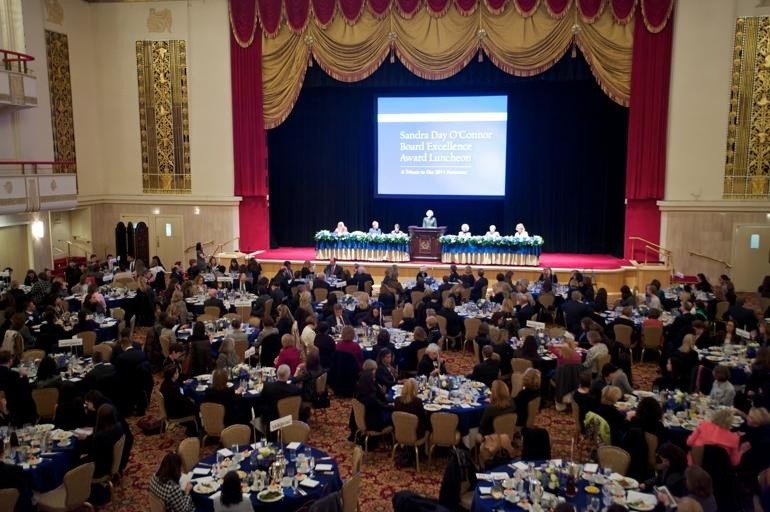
[150,423,362,512]
[1,266,770,452]
[391,453,769,511]
[0,401,133,511]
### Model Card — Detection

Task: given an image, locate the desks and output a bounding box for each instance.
[216,251,246,272]
[440,237,544,266]
[315,235,412,262]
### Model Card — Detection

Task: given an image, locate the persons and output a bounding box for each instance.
[513,223,530,237]
[334,222,348,236]
[485,225,500,238]
[423,210,437,228]
[369,221,381,235]
[391,224,407,236]
[1,244,770,511]
[458,223,472,238]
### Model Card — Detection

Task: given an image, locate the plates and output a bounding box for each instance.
[193,481,221,496]
[257,490,284,503]
[627,500,656,511]
[424,404,442,412]
[614,478,639,490]
